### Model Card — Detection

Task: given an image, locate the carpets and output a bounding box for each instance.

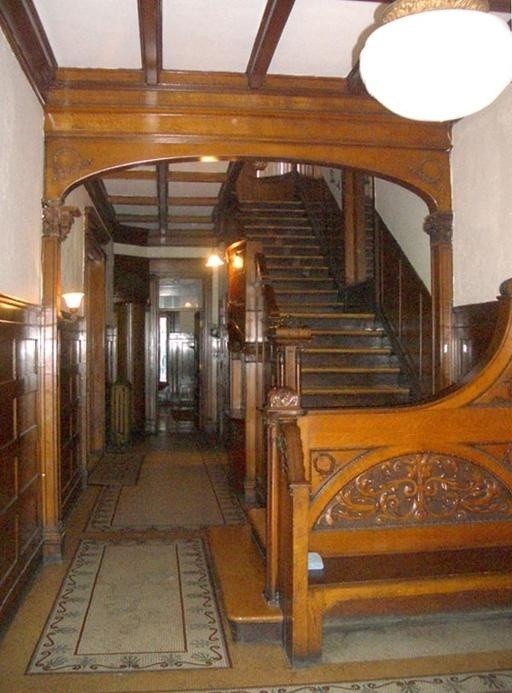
[88,450,147,488]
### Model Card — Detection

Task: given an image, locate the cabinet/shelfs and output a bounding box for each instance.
[165,332,196,421]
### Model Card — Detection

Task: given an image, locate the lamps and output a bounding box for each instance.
[57,292,85,323]
[204,241,224,268]
[358,0,512,124]
